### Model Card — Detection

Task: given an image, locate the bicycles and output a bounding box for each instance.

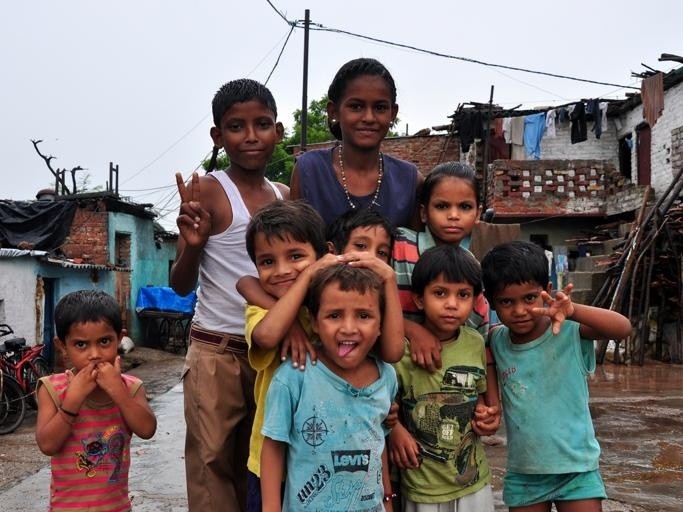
[0,323,52,436]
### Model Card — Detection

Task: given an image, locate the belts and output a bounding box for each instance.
[190,326,247,354]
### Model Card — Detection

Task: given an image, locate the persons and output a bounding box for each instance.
[244,200,406,512]
[288,56,427,225]
[167,79,293,512]
[394,160,503,435]
[483,235,631,512]
[234,208,396,371]
[392,244,495,511]
[34,290,157,507]
[259,263,399,512]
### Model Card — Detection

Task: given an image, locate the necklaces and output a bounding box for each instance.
[338,144,383,211]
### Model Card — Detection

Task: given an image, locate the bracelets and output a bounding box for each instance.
[57,411,76,429]
[59,404,81,418]
[383,492,397,501]
